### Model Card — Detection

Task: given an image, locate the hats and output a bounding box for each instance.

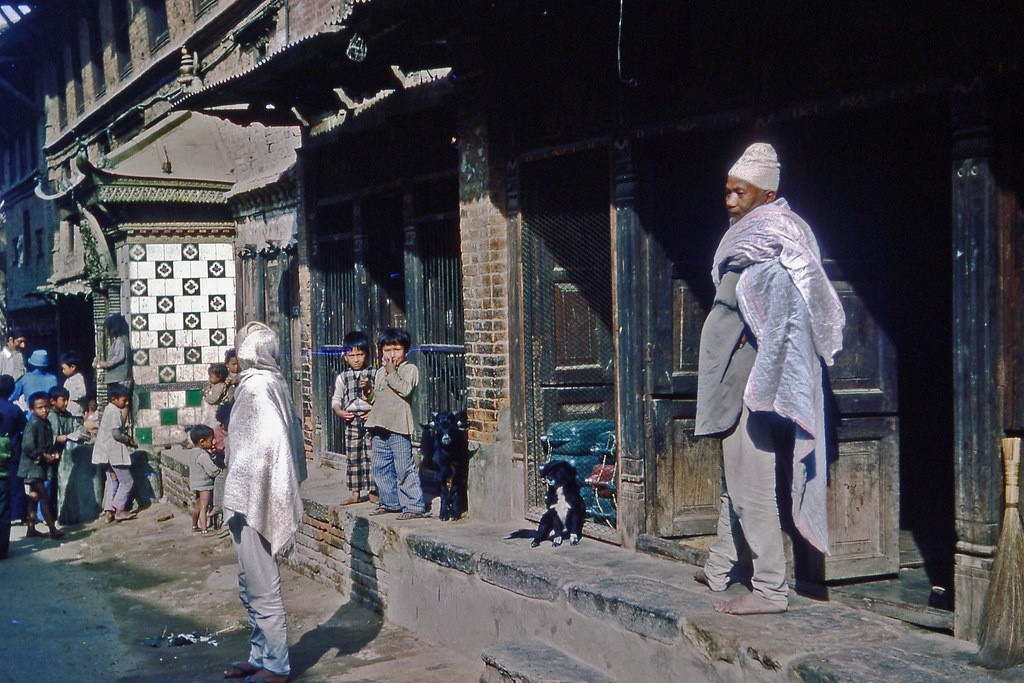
[728,143,781,192]
[27,349,49,367]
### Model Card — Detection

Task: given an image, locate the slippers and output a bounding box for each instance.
[369,495,379,505]
[223,663,257,677]
[338,496,362,506]
[239,673,290,683]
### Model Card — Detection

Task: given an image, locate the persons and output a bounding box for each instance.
[200,363,228,464]
[30,385,98,526]
[225,349,237,383]
[6,349,57,419]
[359,328,426,520]
[83,400,97,421]
[222,320,308,683]
[693,142,846,614]
[0,326,26,560]
[18,391,67,539]
[60,350,89,425]
[92,384,138,523]
[332,332,380,505]
[189,425,220,537]
[97,313,133,389]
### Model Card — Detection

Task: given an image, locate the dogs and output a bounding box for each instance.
[429,410,466,521]
[502,459,586,547]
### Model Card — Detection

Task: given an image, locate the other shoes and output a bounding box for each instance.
[27,530,43,537]
[51,530,65,540]
[191,525,214,537]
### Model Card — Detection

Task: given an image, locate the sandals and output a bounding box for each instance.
[397,511,417,519]
[369,508,390,515]
